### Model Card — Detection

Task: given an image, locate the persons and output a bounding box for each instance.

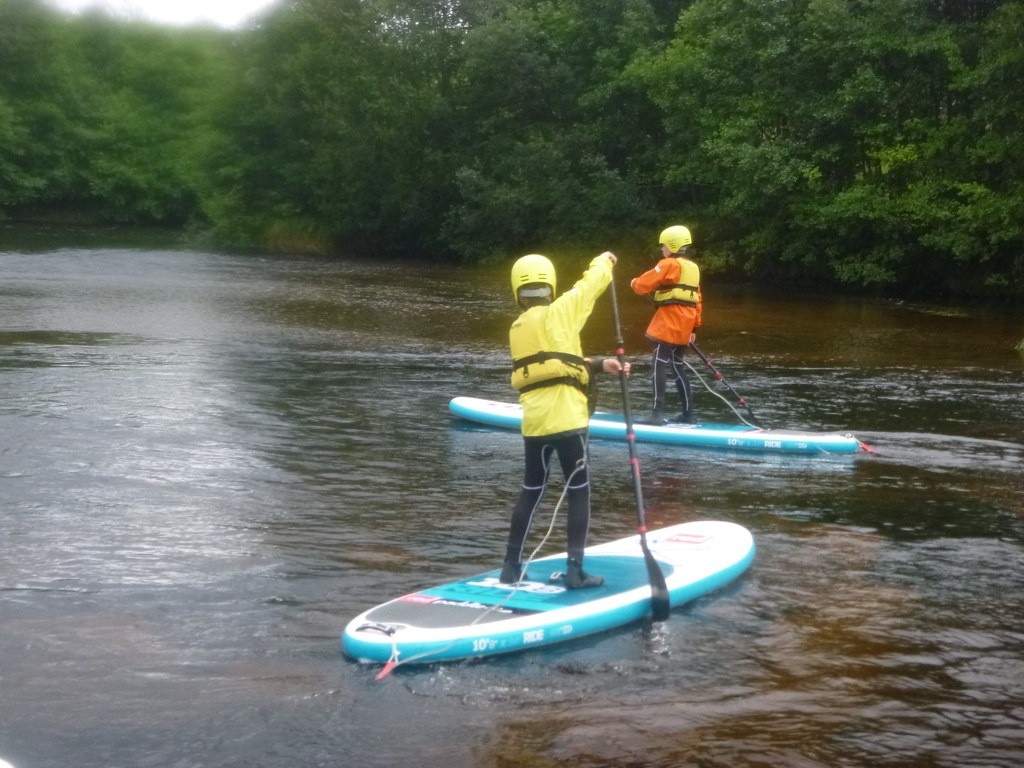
[629,225,703,426]
[499,253,631,589]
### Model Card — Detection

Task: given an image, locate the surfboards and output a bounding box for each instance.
[449,395,862,453]
[341,520,755,665]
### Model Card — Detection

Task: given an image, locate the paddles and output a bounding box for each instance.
[690,343,757,423]
[613,282,672,619]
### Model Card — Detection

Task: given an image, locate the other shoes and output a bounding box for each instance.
[669,414,698,423]
[500,567,527,584]
[633,412,664,426]
[565,572,605,588]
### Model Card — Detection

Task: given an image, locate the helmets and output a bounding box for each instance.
[658,225,693,253]
[511,254,557,305]
